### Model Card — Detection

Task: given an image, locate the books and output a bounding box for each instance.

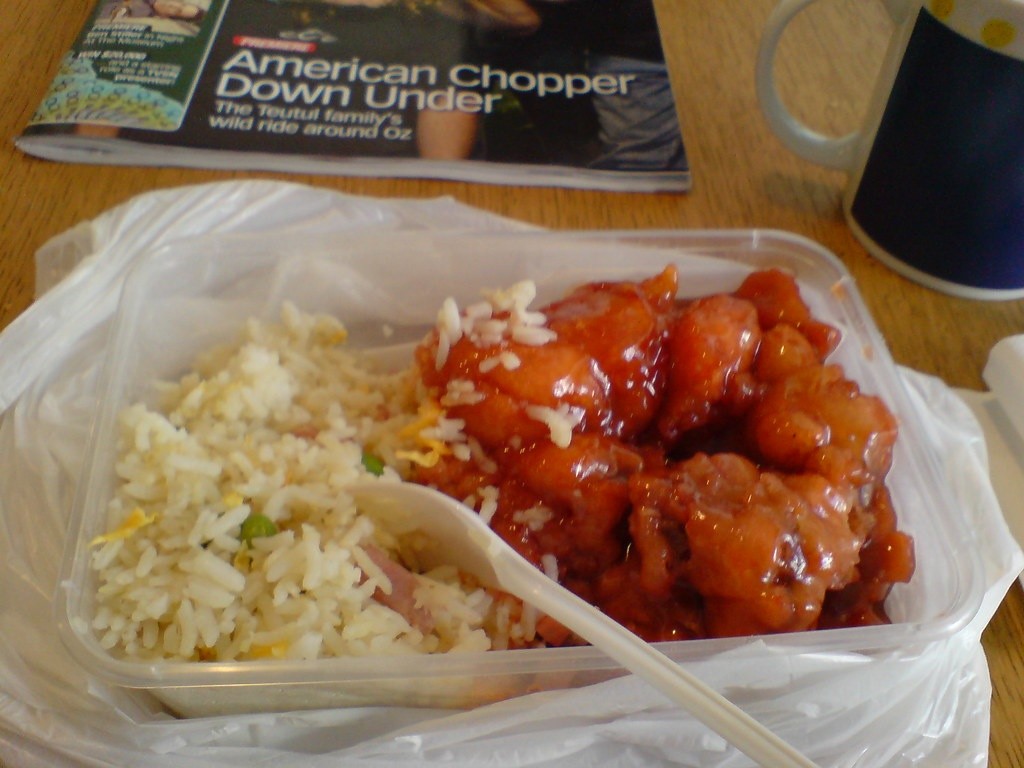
[14,0,698,193]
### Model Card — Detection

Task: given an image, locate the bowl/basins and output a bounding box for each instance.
[57,225,984,719]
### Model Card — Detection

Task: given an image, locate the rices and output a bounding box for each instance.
[82,271,573,711]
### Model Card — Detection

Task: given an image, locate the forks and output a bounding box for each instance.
[354,479,820,767]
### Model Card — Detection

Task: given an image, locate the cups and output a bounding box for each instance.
[755,0,1024,303]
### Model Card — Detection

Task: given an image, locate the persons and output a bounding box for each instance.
[77,0,688,164]
